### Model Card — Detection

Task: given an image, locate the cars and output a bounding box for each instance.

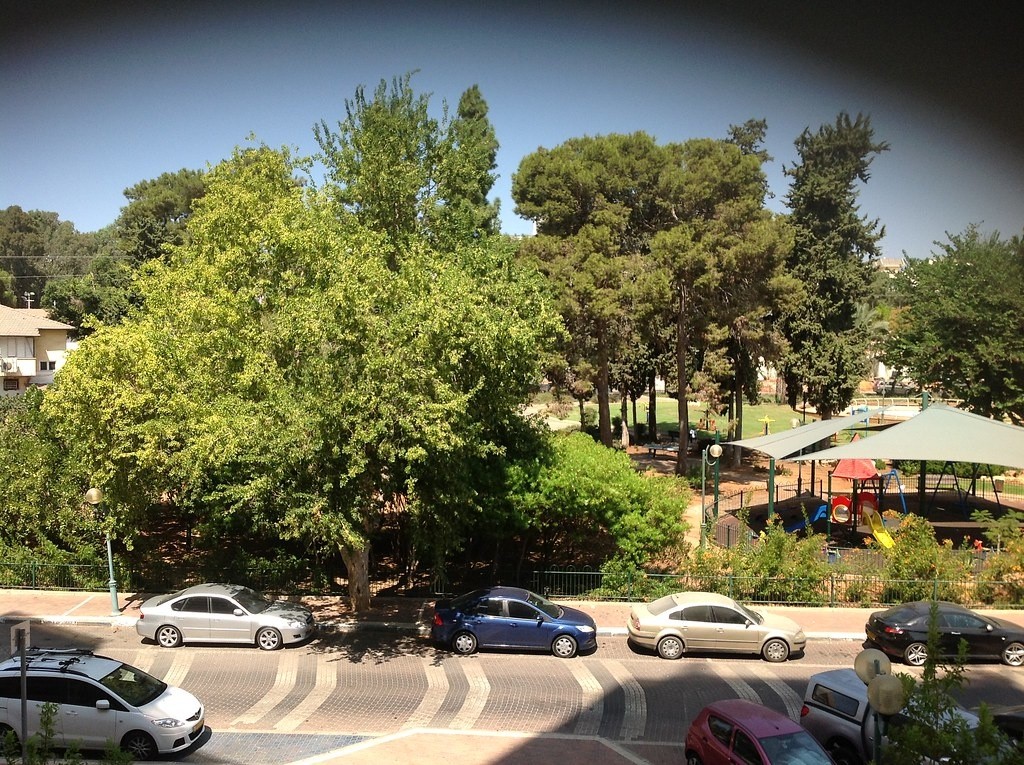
[684,699,838,765]
[431,587,597,658]
[627,592,807,663]
[971,704,1024,750]
[865,601,1024,667]
[800,668,1015,765]
[873,380,913,395]
[135,583,315,650]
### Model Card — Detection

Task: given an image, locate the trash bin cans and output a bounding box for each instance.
[994,479,1004,492]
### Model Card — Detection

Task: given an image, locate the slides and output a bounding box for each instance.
[862,509,898,551]
[783,504,828,534]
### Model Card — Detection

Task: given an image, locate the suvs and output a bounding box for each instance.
[0,648,205,762]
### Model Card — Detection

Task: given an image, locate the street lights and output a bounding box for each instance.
[700,444,723,550]
[853,647,910,765]
[86,488,123,617]
